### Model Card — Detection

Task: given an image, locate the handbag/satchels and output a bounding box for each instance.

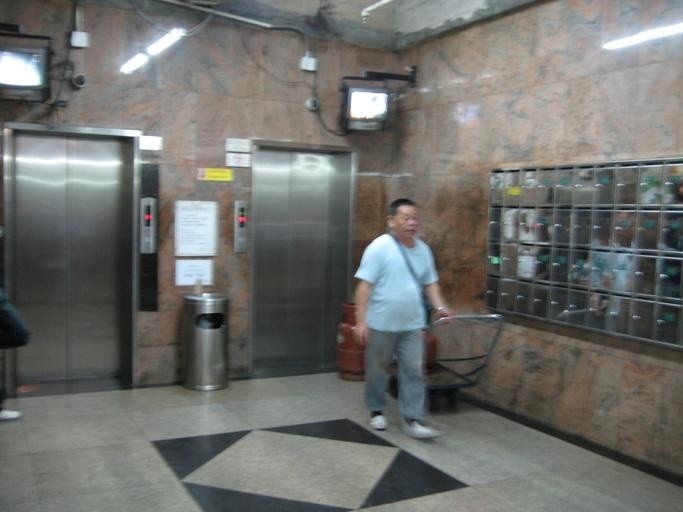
[422,294,433,325]
[0,288,30,349]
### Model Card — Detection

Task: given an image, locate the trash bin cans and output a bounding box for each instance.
[183,295,227,391]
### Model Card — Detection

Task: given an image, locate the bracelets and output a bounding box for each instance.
[434,306,448,310]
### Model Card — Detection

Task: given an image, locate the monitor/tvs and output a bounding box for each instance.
[346,87,392,121]
[0,31,52,103]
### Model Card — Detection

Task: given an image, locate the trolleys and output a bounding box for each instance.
[388,313,504,409]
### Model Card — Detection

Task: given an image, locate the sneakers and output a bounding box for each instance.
[0,409,23,421]
[401,419,442,439]
[368,415,389,430]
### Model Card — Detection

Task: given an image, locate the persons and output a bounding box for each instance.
[580,212,642,329]
[353,198,453,439]
[0,224,30,420]
